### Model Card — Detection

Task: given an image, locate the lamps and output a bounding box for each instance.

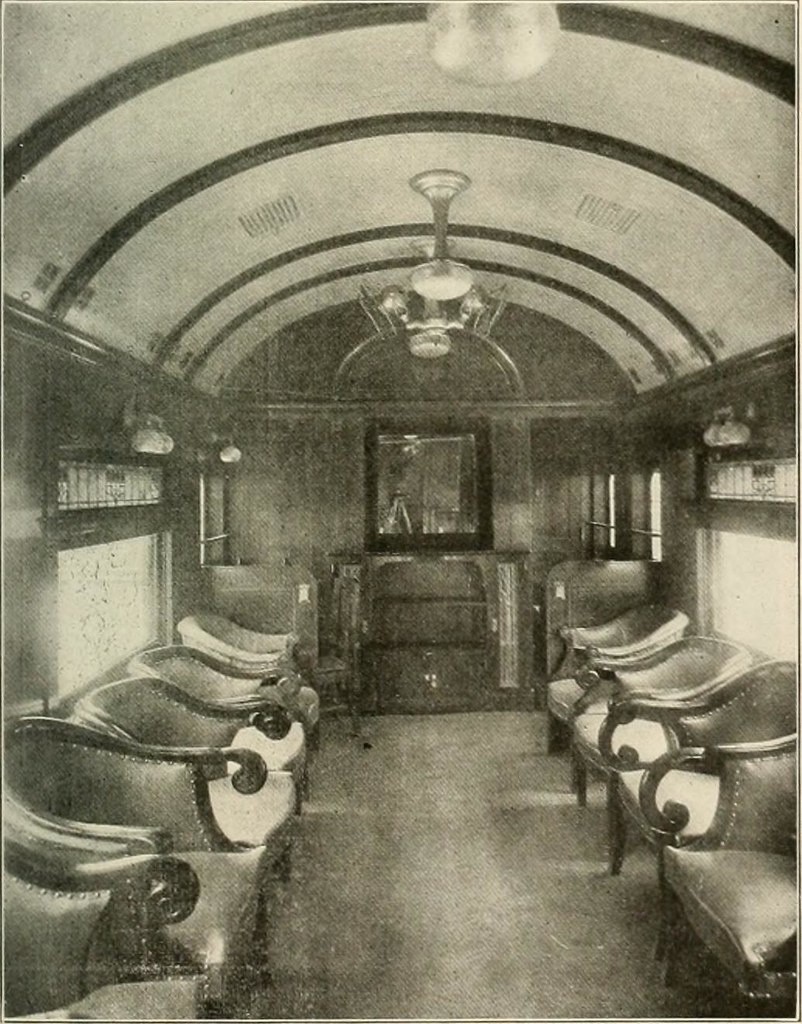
[408,171,478,299]
[222,441,241,461]
[411,300,453,357]
[130,415,174,455]
[426,2,556,85]
[701,412,753,448]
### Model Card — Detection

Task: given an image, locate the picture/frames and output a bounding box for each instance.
[361,419,492,549]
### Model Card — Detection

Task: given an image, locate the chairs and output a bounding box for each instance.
[4,613,316,1019]
[539,606,795,1018]
[315,566,367,735]
